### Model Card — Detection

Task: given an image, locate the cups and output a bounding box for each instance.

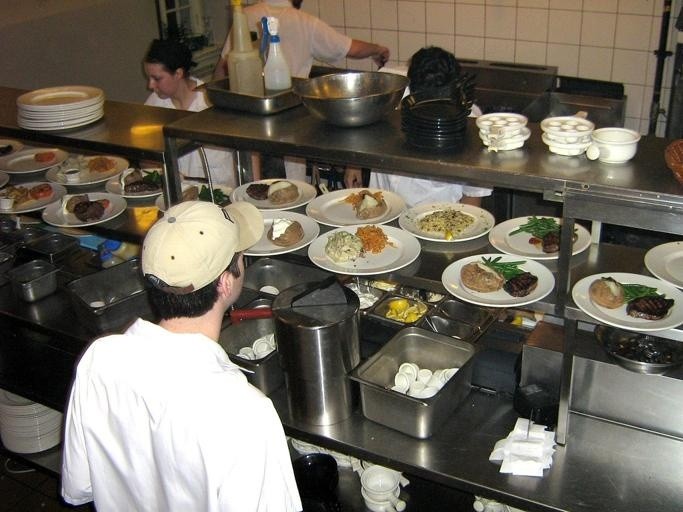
[391,361,457,399]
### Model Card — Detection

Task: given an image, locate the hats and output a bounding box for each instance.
[140,201,265,295]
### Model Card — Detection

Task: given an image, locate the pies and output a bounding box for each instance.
[421,210,474,237]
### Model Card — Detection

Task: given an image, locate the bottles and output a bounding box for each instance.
[97,238,138,266]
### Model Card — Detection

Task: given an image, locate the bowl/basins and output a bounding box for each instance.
[293,70,410,129]
[594,324,678,375]
[238,332,274,360]
[590,127,641,167]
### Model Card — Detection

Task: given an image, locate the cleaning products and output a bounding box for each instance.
[260,15,293,98]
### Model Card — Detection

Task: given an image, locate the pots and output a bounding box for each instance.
[288,436,408,511]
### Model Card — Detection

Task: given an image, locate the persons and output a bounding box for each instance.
[212,1,390,180]
[142,37,262,188]
[60,198,304,512]
[343,45,494,209]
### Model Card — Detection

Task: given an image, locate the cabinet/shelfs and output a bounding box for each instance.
[0,88,241,479]
[163,106,683,512]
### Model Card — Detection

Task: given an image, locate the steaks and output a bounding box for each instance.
[125,179,160,195]
[74,201,104,222]
[543,231,560,253]
[246,184,269,200]
[626,296,674,321]
[503,272,538,297]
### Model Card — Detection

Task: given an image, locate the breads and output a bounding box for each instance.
[589,277,625,308]
[356,194,387,220]
[61,194,89,213]
[268,181,298,204]
[326,231,362,264]
[461,261,503,292]
[267,219,304,247]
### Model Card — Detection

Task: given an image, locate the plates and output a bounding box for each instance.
[640,241,681,287]
[477,111,529,150]
[45,156,129,187]
[154,182,234,214]
[12,85,104,131]
[0,392,61,455]
[226,209,322,260]
[230,177,318,214]
[306,186,407,230]
[42,192,126,230]
[346,278,453,327]
[402,199,496,246]
[5,227,146,326]
[571,274,681,332]
[400,96,467,156]
[441,253,556,311]
[537,115,591,161]
[307,224,420,274]
[3,150,67,176]
[1,180,67,218]
[104,166,183,198]
[1,139,24,160]
[487,216,593,262]
